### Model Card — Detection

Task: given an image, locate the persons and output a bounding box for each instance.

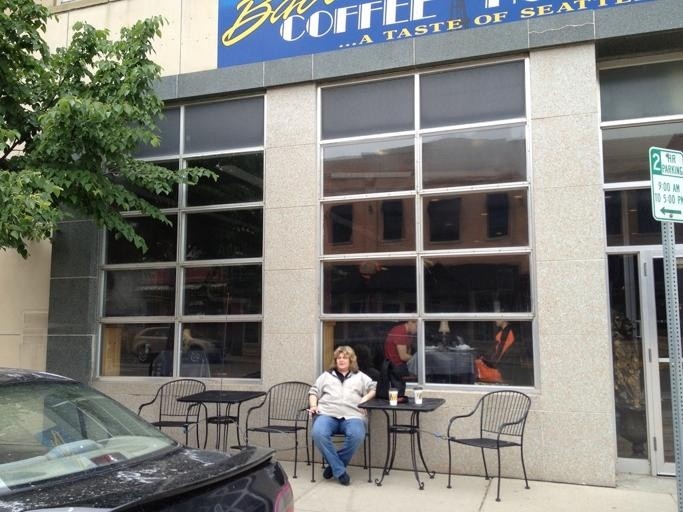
[475,320,515,380]
[383,321,418,383]
[307,346,378,485]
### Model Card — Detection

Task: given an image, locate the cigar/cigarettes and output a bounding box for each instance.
[305,408,315,414]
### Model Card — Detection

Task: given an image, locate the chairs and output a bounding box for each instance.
[245,380,310,479]
[446,390,532,502]
[310,380,372,483]
[136,379,206,450]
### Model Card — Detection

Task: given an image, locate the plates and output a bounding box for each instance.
[449,347,474,351]
[424,346,439,352]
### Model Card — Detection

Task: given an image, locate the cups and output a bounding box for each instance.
[413,387,423,404]
[388,388,398,406]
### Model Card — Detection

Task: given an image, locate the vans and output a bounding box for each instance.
[131,326,227,363]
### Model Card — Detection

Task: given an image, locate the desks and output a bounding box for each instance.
[358,396,446,490]
[177,389,267,451]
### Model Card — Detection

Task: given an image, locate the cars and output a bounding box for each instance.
[0,367,293,512]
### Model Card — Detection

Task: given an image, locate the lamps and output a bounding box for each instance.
[439,321,450,345]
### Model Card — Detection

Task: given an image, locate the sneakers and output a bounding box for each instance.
[340,473,350,483]
[323,466,333,478]
